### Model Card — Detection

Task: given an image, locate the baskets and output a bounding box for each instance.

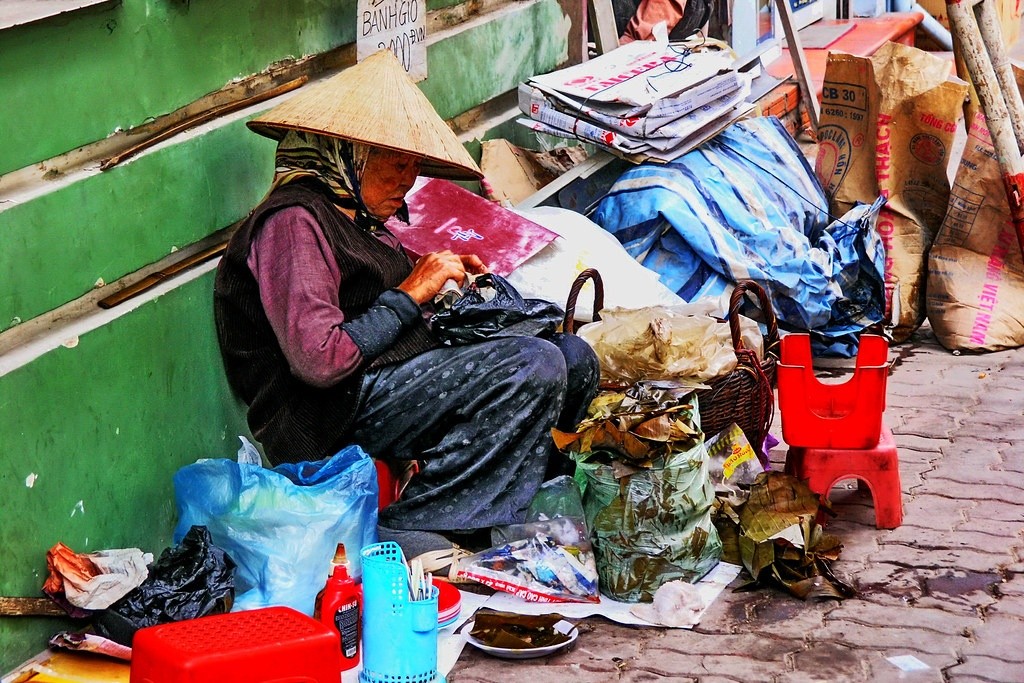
[356,539,447,683]
[563,267,780,466]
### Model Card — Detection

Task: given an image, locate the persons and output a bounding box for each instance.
[213,47,605,591]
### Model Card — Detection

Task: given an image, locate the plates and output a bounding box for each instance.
[458,619,579,658]
[426,578,461,627]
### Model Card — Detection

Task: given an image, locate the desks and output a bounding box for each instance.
[765,8,925,124]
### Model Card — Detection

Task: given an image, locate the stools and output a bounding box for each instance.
[801,421,903,530]
[129,607,341,682]
[778,332,889,448]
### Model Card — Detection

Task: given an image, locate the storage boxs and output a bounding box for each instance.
[359,542,447,683]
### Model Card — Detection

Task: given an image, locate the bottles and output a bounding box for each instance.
[312,543,362,673]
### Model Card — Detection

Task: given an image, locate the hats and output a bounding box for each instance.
[242,47,488,183]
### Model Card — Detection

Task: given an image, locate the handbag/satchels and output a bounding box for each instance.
[171,435,380,619]
[430,273,566,347]
[569,387,727,604]
[91,525,238,649]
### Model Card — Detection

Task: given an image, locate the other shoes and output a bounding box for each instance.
[405,540,490,595]
